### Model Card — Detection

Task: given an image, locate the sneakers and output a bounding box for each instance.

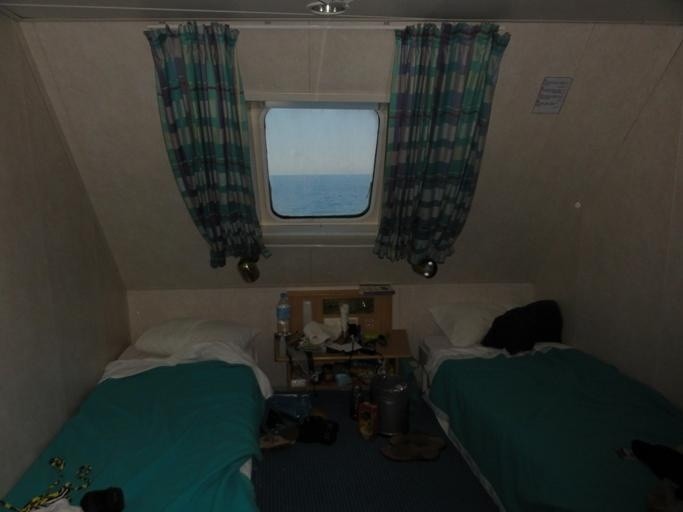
[388,433,446,452]
[378,443,440,462]
[296,416,340,444]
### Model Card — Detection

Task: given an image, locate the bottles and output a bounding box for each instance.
[276,292,291,334]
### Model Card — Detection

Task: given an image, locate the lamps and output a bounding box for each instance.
[238,254,260,284]
[408,255,439,279]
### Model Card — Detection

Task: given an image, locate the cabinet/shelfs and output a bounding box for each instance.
[269,289,416,421]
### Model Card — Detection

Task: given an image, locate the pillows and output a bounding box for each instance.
[425,299,508,347]
[132,316,261,359]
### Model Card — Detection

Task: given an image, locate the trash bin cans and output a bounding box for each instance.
[370,375,409,436]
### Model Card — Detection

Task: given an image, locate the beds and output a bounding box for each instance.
[0,343,267,512]
[413,337,683,512]
